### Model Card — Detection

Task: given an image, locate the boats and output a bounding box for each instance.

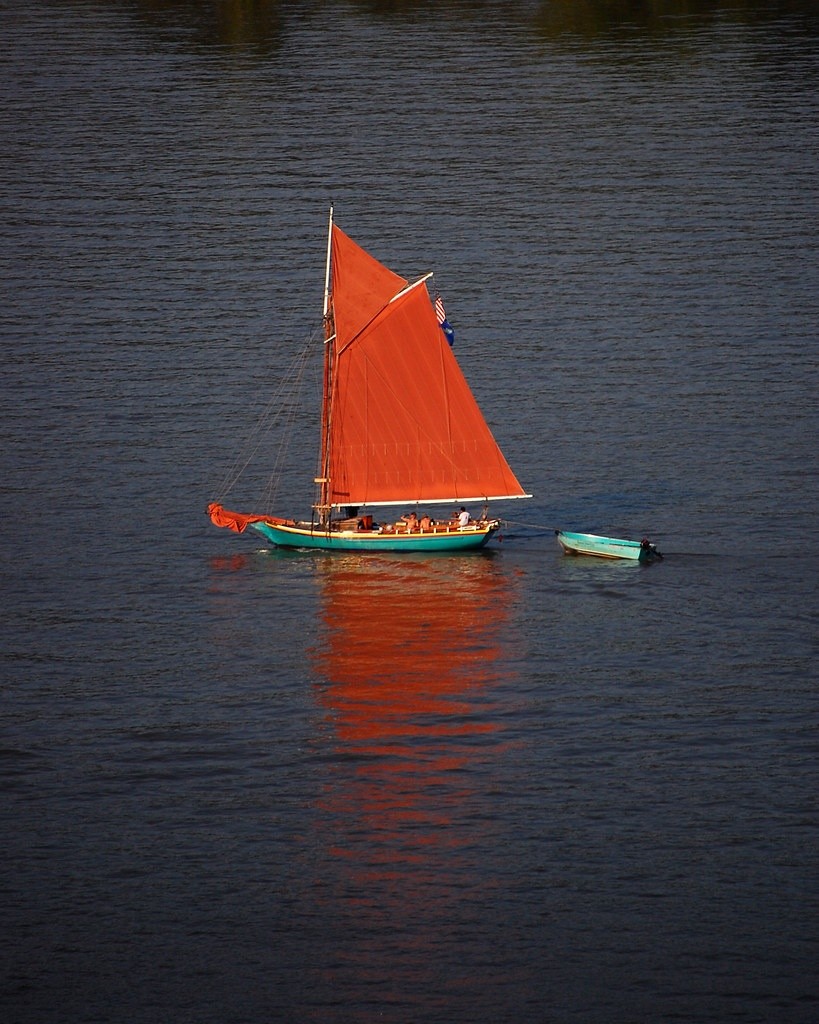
[557,530,657,561]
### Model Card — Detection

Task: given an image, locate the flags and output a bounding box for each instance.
[434,296,455,347]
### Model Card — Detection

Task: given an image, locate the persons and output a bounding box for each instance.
[401,512,418,530]
[453,506,470,525]
[345,506,360,518]
[419,512,431,529]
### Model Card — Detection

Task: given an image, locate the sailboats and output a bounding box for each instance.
[207,202,534,551]
